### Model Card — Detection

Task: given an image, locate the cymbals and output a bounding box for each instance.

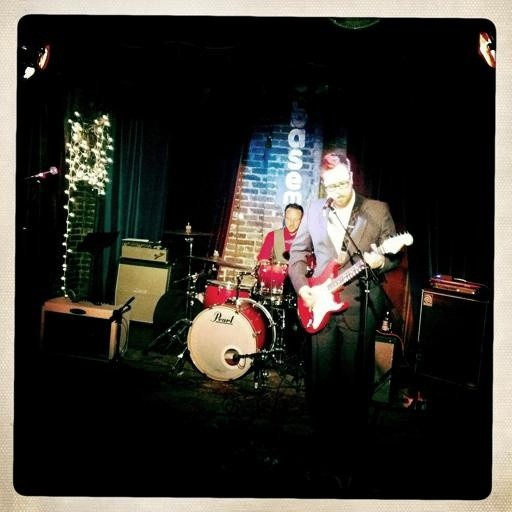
[199,256,244,269]
[169,232,213,237]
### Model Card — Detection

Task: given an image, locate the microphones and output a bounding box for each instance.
[321,193,338,216]
[28,166,58,181]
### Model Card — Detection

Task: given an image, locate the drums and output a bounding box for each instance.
[204,280,250,309]
[258,262,289,288]
[186,298,277,383]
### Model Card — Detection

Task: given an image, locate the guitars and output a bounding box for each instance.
[297,233,413,335]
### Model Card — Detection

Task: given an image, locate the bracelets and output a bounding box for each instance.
[379,261,384,271]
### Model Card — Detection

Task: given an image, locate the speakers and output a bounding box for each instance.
[413,287,493,391]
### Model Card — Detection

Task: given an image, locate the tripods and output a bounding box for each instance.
[143,241,203,372]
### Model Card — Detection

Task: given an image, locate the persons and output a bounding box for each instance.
[287,152,403,427]
[256,203,315,274]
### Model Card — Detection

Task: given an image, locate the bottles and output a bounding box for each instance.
[185,223,191,234]
[381,311,393,333]
